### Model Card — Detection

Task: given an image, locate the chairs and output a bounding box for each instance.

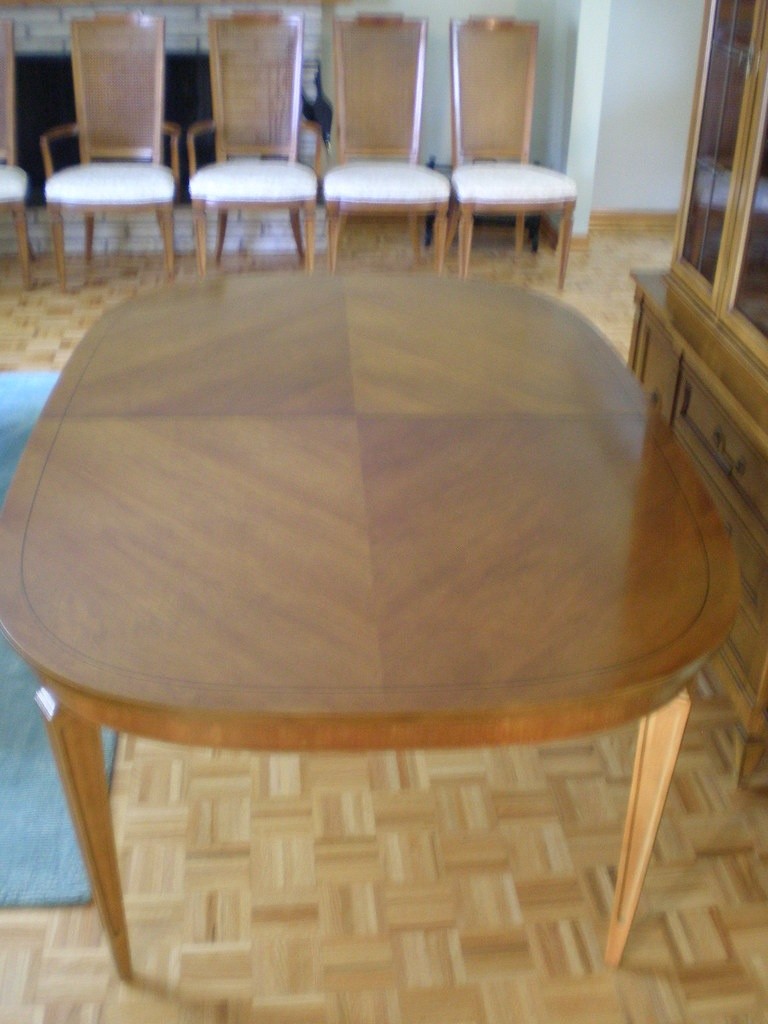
[186,11,322,275]
[325,10,451,272]
[40,14,182,290]
[451,15,579,293]
[0,18,39,286]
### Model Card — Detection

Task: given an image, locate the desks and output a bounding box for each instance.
[1,267,738,982]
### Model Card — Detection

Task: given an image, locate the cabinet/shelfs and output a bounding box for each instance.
[624,0,766,790]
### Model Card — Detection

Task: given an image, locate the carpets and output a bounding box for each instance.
[0,365,114,911]
